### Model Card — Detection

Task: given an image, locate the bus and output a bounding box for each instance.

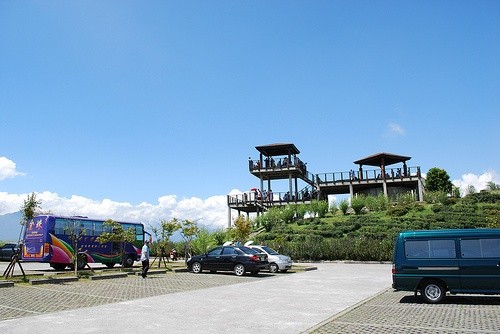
[21,215,152,271]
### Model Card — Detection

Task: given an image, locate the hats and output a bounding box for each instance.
[144,239,149,243]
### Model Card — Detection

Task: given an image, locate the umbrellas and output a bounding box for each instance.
[244,241,254,247]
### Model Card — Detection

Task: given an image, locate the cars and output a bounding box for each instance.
[0,243,22,261]
[186,245,268,277]
[245,245,292,273]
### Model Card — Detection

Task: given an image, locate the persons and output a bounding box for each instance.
[170,247,195,261]
[140,240,150,279]
[223,241,232,246]
[244,157,419,202]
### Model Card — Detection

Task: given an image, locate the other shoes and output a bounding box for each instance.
[141,273,145,278]
[142,275,147,277]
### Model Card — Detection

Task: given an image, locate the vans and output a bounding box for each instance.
[391,227,500,304]
